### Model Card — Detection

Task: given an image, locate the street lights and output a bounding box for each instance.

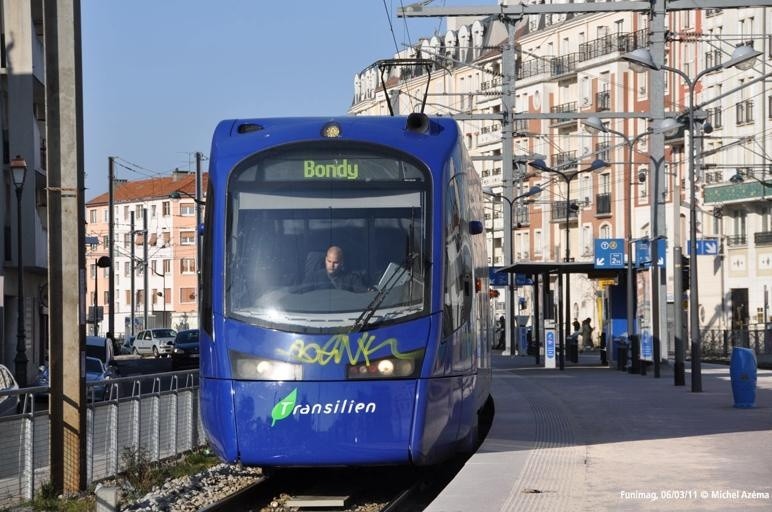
[167,187,206,207]
[578,113,684,369]
[480,183,541,360]
[620,43,761,389]
[93,255,112,338]
[528,155,612,360]
[8,154,33,386]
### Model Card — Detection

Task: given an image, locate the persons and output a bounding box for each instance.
[298,245,374,293]
[572,318,580,332]
[495,316,505,349]
[579,317,595,353]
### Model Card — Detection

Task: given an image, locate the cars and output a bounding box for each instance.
[172,328,200,367]
[35,356,114,401]
[1,363,22,415]
[131,327,177,358]
[84,335,115,364]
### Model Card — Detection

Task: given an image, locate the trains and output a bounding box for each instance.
[202,110,496,476]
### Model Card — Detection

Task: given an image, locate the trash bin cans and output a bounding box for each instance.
[628,335,640,374]
